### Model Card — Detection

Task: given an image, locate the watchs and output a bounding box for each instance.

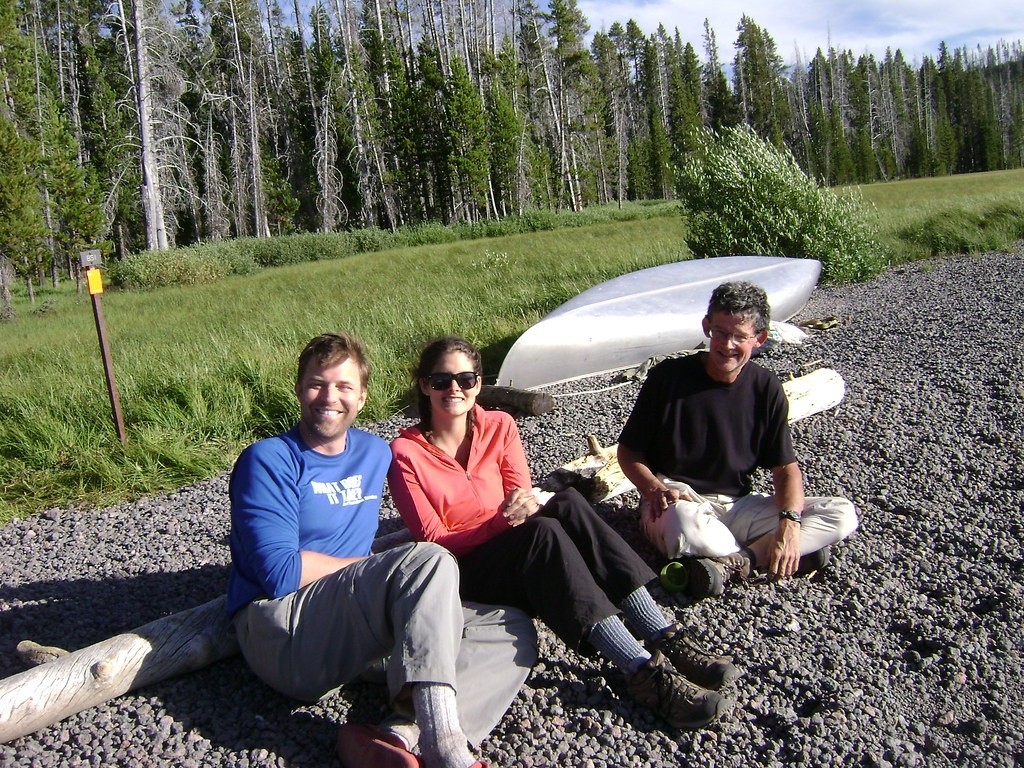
[779,510,802,524]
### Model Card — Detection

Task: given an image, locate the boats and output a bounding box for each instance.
[495,255,824,387]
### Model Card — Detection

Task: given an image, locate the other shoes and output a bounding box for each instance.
[469,759,488,768]
[337,724,419,768]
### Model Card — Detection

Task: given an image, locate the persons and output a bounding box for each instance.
[224,334,537,768]
[618,282,858,598]
[386,337,734,730]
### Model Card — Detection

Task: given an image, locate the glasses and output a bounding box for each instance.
[426,371,479,390]
[707,325,758,343]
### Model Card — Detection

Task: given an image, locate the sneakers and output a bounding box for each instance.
[645,623,737,691]
[689,552,750,599]
[798,546,829,572]
[621,649,726,729]
